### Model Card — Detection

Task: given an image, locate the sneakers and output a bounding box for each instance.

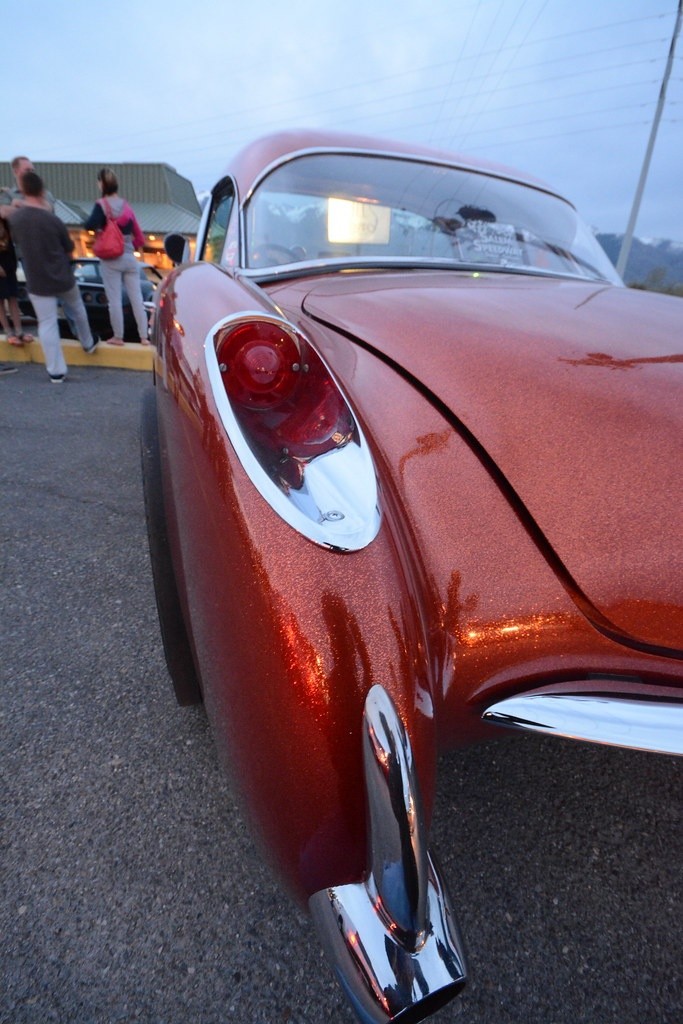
[84,335,100,354]
[51,374,66,383]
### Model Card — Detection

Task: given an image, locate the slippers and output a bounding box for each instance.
[6,333,34,345]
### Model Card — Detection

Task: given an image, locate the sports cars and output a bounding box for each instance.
[138,129,683,1023]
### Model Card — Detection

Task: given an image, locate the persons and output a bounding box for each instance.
[83,167,154,345]
[0,200,34,348]
[9,156,57,213]
[8,172,100,383]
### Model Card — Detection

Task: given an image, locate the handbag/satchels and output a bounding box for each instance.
[93,196,124,259]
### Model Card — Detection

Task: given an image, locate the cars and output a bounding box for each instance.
[17,259,164,338]
